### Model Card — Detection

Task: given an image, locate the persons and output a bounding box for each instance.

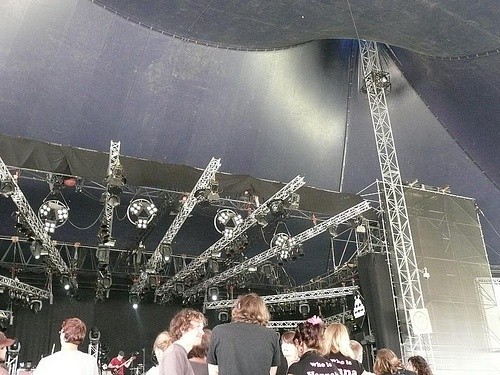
[33,318,99,375]
[287,315,341,375]
[146,323,433,375]
[160,309,207,375]
[0,331,15,375]
[108,351,132,375]
[206,293,281,375]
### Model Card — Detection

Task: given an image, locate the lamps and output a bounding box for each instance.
[0,165,368,357]
[359,71,391,97]
